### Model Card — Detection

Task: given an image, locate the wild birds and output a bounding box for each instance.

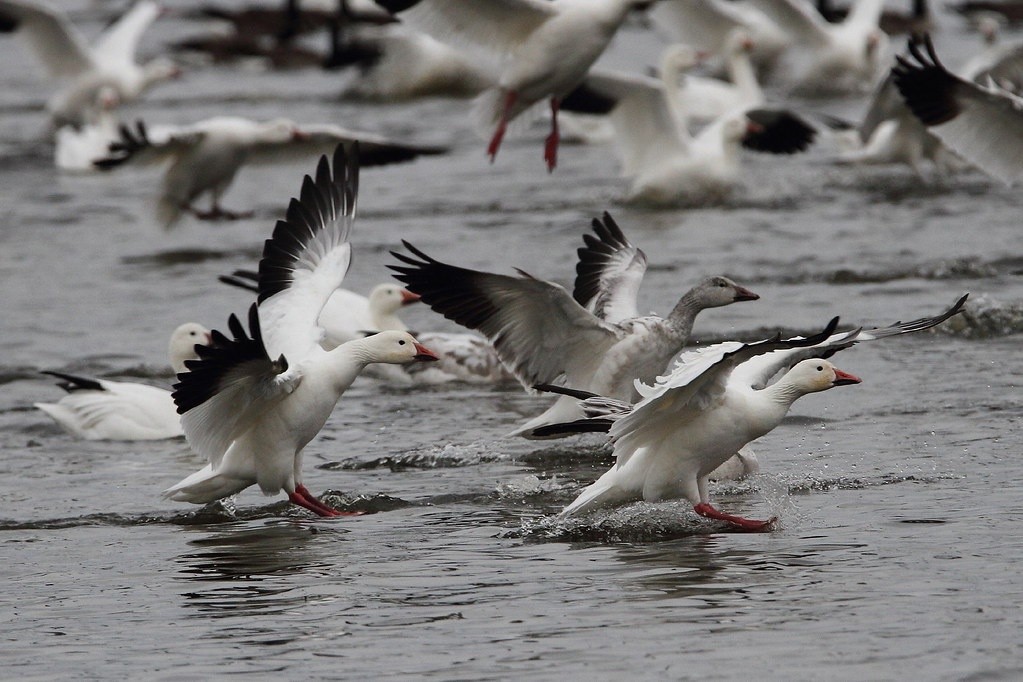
[0,0,1022,530]
